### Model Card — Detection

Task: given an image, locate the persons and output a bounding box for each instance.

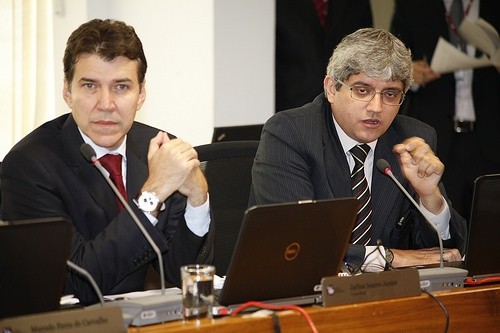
[249,27,467,272]
[326,0,500,219]
[0,19,211,305]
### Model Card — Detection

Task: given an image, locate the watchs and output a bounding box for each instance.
[135,191,166,215]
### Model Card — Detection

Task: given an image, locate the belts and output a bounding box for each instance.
[454,120,477,132]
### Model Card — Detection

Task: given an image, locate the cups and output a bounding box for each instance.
[180,265,216,324]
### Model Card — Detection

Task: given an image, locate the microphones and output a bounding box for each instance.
[376,159,469,291]
[79,144,184,326]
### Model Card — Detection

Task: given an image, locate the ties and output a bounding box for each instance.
[98,153,128,212]
[449,0,466,53]
[349,143,372,246]
[313,0,329,31]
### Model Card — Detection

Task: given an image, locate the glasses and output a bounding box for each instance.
[335,79,406,106]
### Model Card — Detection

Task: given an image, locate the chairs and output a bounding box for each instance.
[192,140,261,277]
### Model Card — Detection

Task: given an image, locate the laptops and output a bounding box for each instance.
[426,173,500,276]
[215,197,360,313]
[0,218,74,320]
